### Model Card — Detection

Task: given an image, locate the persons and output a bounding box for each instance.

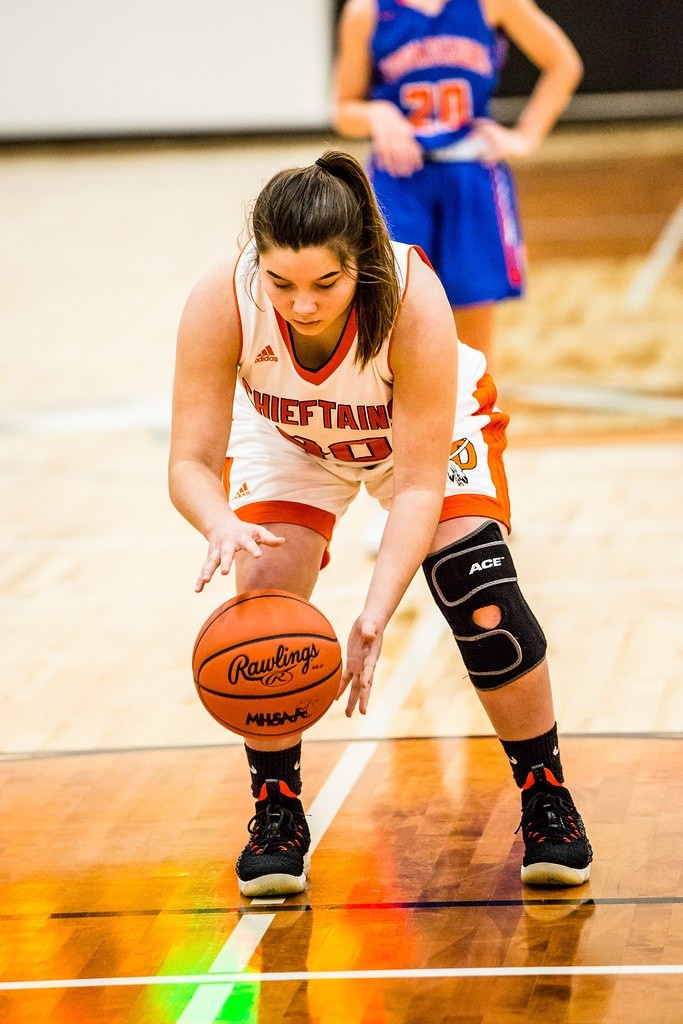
[167,149,595,901]
[330,0,584,373]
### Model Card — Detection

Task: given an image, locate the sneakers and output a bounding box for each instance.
[520,765,594,887]
[237,778,313,895]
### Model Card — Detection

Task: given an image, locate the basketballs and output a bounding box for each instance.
[193,587,343,739]
